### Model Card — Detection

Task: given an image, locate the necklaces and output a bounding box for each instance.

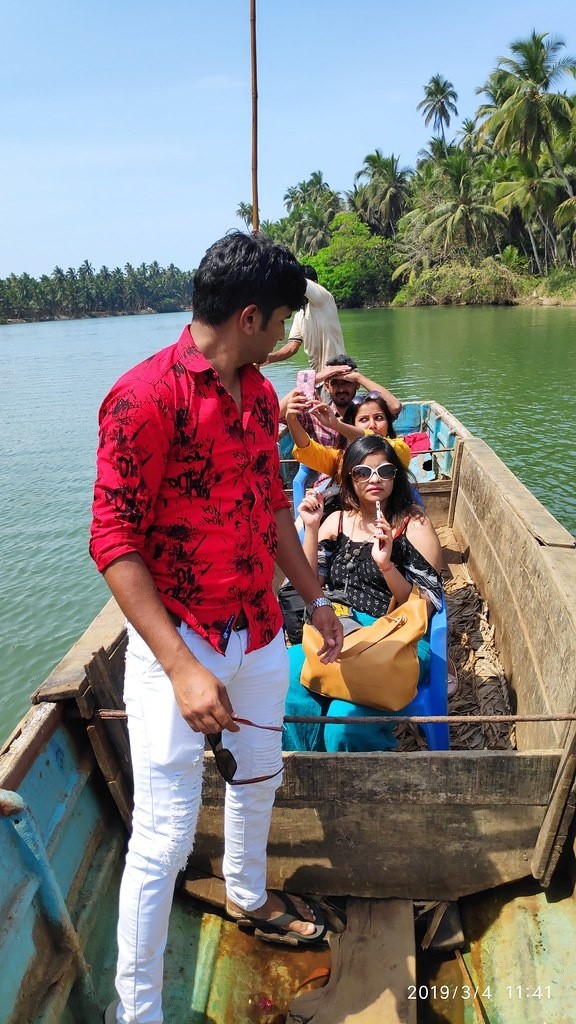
[342,512,388,595]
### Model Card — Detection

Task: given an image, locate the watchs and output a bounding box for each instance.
[303,597,336,619]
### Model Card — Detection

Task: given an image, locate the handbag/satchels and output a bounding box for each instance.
[303,485,342,531]
[299,583,429,713]
[277,580,309,645]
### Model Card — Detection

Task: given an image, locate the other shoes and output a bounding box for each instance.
[448,658,458,697]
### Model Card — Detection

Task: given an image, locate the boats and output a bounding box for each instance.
[0,399,576,1024]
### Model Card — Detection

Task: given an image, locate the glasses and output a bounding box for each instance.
[348,462,398,483]
[351,390,382,405]
[205,716,295,786]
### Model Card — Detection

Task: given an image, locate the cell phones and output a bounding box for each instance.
[375,501,382,550]
[296,371,317,406]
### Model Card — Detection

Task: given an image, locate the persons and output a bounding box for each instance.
[273,356,446,753]
[251,264,347,394]
[88,234,325,1024]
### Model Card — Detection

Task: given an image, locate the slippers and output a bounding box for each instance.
[100,997,121,1023]
[225,888,329,946]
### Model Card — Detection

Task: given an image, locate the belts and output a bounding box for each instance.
[165,608,248,632]
